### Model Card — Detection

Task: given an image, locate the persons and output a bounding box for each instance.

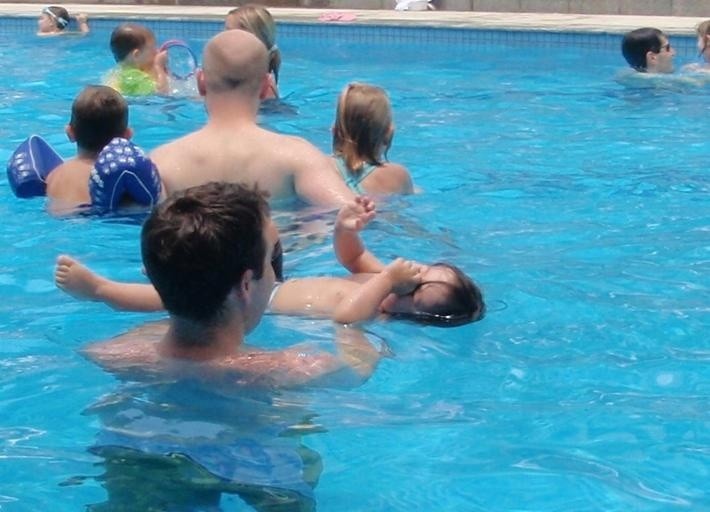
[681,21,710,77]
[77,180,388,512]
[145,29,362,249]
[620,26,699,102]
[37,6,89,39]
[10,85,160,225]
[55,200,484,330]
[103,23,174,101]
[322,81,426,240]
[224,4,294,116]
